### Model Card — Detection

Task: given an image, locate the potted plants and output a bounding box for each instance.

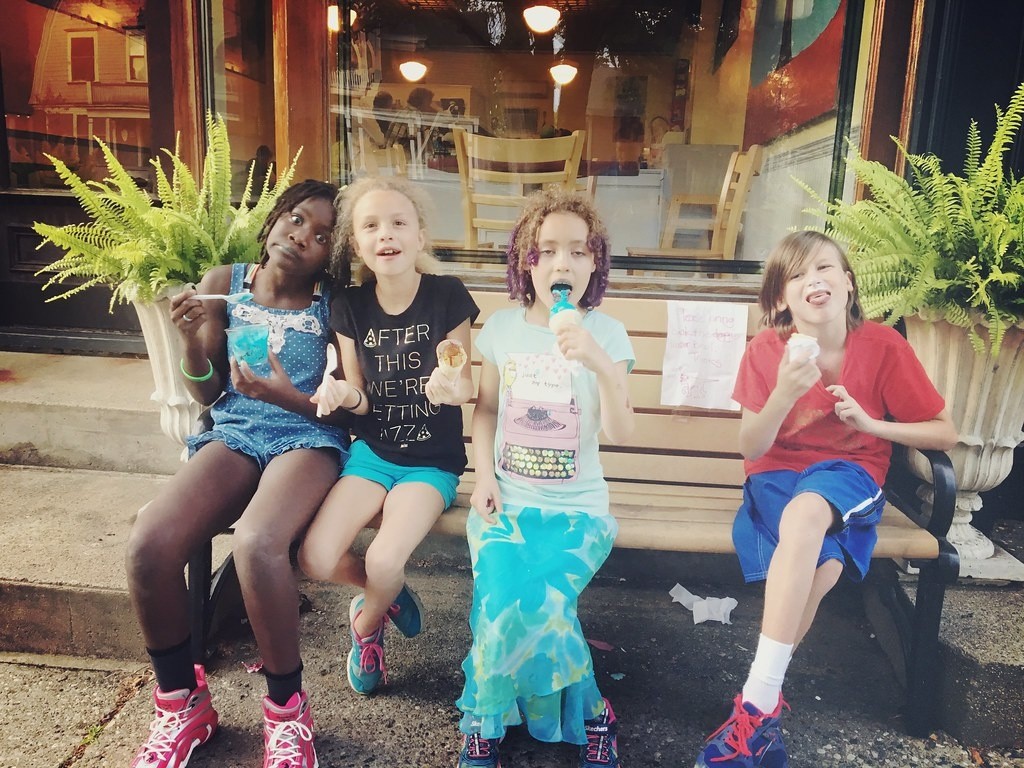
[790,79,1023,560]
[28,112,304,455]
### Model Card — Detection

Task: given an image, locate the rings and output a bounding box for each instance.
[183,315,193,322]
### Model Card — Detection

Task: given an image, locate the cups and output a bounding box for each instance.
[224,324,269,381]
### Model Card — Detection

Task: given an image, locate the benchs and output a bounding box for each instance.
[174,276,961,737]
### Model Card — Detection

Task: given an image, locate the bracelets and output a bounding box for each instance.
[346,389,362,410]
[180,355,213,383]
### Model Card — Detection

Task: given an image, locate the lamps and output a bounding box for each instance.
[327,1,580,84]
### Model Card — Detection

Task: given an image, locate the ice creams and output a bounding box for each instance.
[226,327,269,365]
[549,288,581,335]
[435,339,468,383]
[237,293,253,303]
[787,333,816,362]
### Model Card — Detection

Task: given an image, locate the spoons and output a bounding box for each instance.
[315,344,338,418]
[171,292,254,305]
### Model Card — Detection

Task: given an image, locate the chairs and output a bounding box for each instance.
[448,127,585,267]
[625,141,761,278]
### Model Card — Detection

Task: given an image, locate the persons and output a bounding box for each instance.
[691,228,956,768]
[123,177,352,767]
[296,167,483,699]
[454,192,638,768]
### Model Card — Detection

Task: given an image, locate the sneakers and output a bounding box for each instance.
[262,690,319,768]
[131,663,218,768]
[693,691,791,768]
[387,584,425,637]
[578,696,620,768]
[347,593,390,695]
[457,727,507,768]
